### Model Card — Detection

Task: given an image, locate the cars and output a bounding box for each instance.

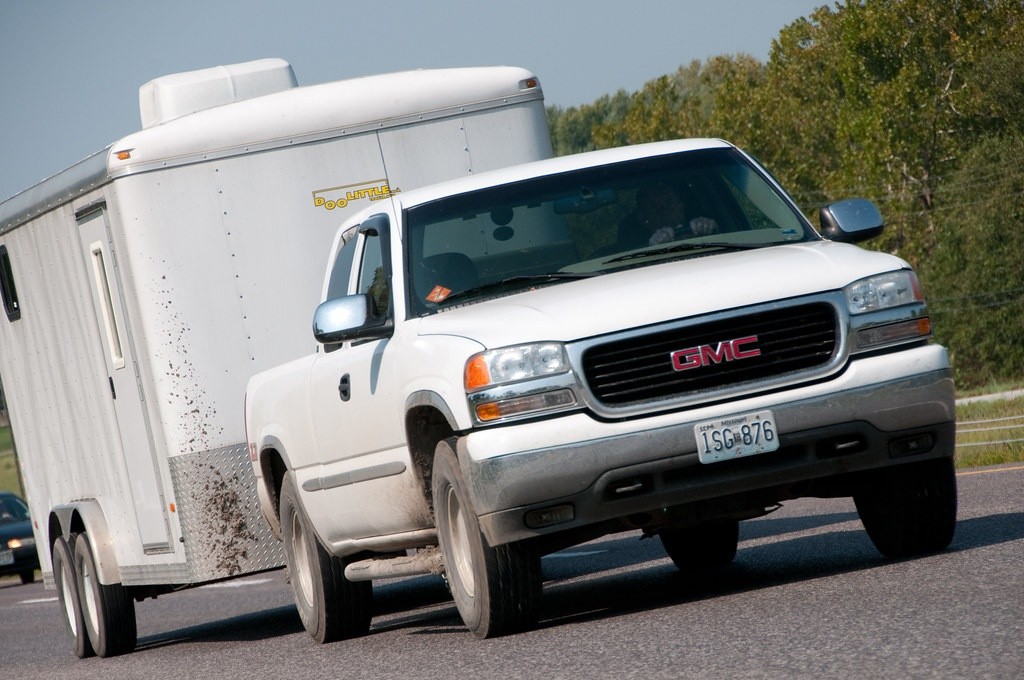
[0,490,41,583]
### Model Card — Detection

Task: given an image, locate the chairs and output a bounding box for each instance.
[423,251,480,303]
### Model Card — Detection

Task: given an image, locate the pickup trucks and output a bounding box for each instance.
[243,137,956,645]
